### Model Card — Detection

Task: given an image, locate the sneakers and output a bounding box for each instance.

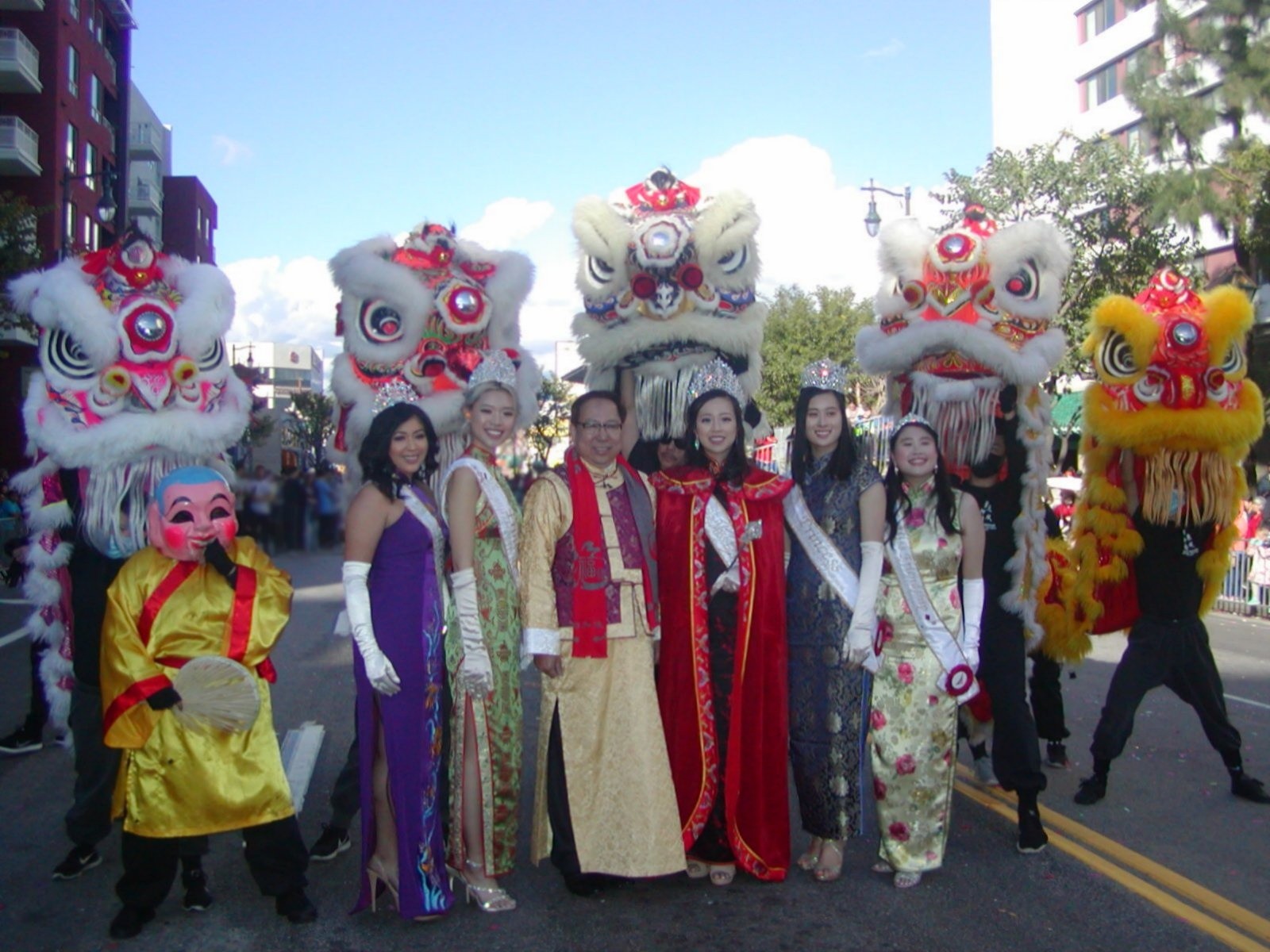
[55,735,67,745]
[313,822,353,862]
[51,850,102,881]
[0,728,45,752]
[182,872,212,911]
[1016,799,1049,852]
[1046,740,1067,767]
[1075,773,1107,805]
[1230,776,1270,805]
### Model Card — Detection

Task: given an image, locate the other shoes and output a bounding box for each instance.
[551,846,602,896]
[276,887,317,925]
[1248,599,1261,606]
[111,902,154,937]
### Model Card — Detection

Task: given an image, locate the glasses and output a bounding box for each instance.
[573,419,622,434]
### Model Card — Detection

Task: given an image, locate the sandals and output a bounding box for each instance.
[815,834,841,882]
[685,857,707,877]
[895,866,925,888]
[871,860,894,873]
[797,834,823,872]
[710,864,735,887]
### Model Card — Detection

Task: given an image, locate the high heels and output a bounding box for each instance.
[446,862,465,892]
[461,858,517,912]
[365,856,444,921]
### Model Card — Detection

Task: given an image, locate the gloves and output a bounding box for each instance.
[342,561,401,697]
[961,577,983,673]
[842,540,885,673]
[449,568,494,698]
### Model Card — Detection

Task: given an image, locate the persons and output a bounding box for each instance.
[100,466,319,937]
[869,412,984,889]
[343,402,455,920]
[0,532,65,753]
[438,348,687,914]
[647,356,886,882]
[956,733,1000,786]
[232,458,343,554]
[1075,442,1270,804]
[53,468,213,909]
[1225,496,1270,619]
[619,362,696,472]
[1028,500,1070,766]
[308,673,452,859]
[945,383,1049,851]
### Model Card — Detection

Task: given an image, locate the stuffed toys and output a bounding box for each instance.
[1021,265,1264,660]
[327,218,543,511]
[570,163,765,440]
[855,201,1073,649]
[7,221,254,760]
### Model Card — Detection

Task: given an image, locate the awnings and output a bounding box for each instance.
[1051,391,1086,433]
